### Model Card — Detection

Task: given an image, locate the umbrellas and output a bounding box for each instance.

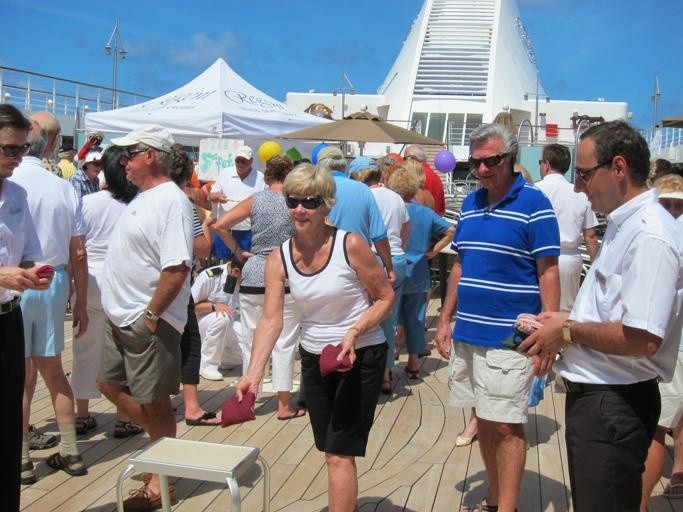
[275,104,447,158]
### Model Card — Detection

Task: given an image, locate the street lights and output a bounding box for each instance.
[44,96,52,113]
[649,72,660,139]
[4,90,12,104]
[522,69,550,142]
[332,71,355,157]
[81,102,90,121]
[101,15,126,108]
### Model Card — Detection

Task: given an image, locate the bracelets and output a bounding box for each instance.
[349,327,360,334]
[142,309,159,320]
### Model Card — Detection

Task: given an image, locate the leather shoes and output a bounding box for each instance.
[122,479,179,511]
[456,428,478,446]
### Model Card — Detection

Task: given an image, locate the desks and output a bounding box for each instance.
[435,242,460,312]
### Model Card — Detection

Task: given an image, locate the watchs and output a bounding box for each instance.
[560,317,578,345]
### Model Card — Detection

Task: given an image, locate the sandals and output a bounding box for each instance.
[22,415,146,484]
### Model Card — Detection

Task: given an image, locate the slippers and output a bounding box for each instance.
[404,368,419,380]
[381,371,392,396]
[463,496,500,512]
[664,482,683,498]
[185,412,223,425]
[277,408,305,420]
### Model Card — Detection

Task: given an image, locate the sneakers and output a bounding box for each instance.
[199,367,223,381]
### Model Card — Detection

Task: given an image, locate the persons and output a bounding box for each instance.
[636,215,683,511]
[2,88,682,487]
[226,163,396,512]
[92,123,196,512]
[432,121,561,511]
[515,119,682,511]
[0,106,56,511]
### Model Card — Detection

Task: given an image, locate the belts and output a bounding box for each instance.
[564,379,593,394]
[0,296,21,313]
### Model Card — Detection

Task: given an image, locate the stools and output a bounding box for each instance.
[117,437,272,512]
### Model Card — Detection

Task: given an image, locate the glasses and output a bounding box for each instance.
[469,154,508,167]
[286,195,323,209]
[576,163,606,178]
[122,148,150,159]
[1,144,30,157]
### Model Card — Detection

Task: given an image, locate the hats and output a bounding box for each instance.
[111,123,175,154]
[348,156,379,178]
[235,145,253,160]
[81,152,100,165]
[317,146,344,160]
